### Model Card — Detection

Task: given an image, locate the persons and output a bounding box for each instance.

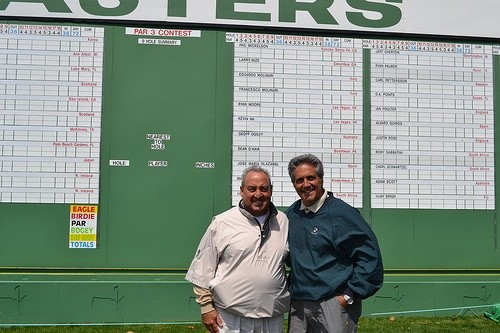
[283,154,384,333]
[184,166,291,333]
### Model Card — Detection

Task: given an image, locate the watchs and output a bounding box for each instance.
[343,294,354,304]
